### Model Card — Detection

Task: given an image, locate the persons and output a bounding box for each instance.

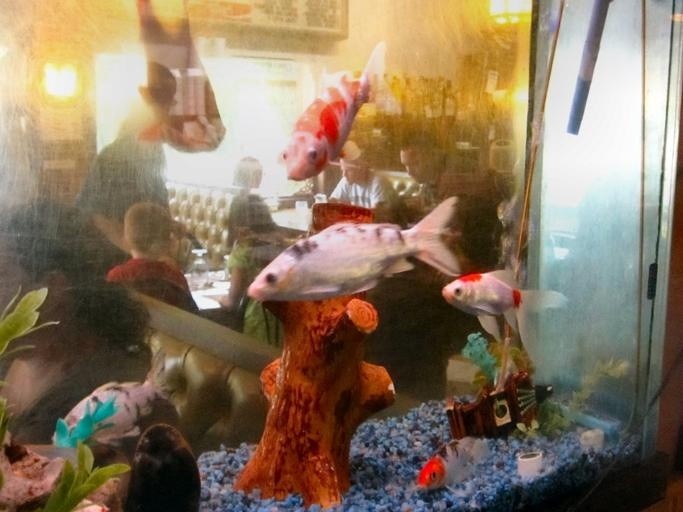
[103,199,199,315]
[206,193,295,350]
[224,154,279,252]
[394,132,506,236]
[76,106,172,257]
[0,198,153,446]
[326,144,401,227]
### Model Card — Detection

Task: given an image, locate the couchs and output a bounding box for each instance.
[98,169,462,466]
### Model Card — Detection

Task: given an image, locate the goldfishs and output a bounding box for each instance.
[442,269,569,363]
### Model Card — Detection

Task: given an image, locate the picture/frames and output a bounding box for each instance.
[184,0,348,38]
